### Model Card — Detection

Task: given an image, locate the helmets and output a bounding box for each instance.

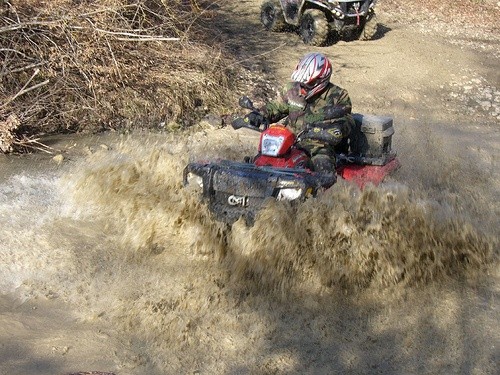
[291,52,332,101]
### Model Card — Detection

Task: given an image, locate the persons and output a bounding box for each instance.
[243,53,356,175]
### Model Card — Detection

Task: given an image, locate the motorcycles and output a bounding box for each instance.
[182,97,402,228]
[261,0,378,46]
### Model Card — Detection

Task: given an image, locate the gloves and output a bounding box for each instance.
[243,112,264,126]
[321,128,343,144]
